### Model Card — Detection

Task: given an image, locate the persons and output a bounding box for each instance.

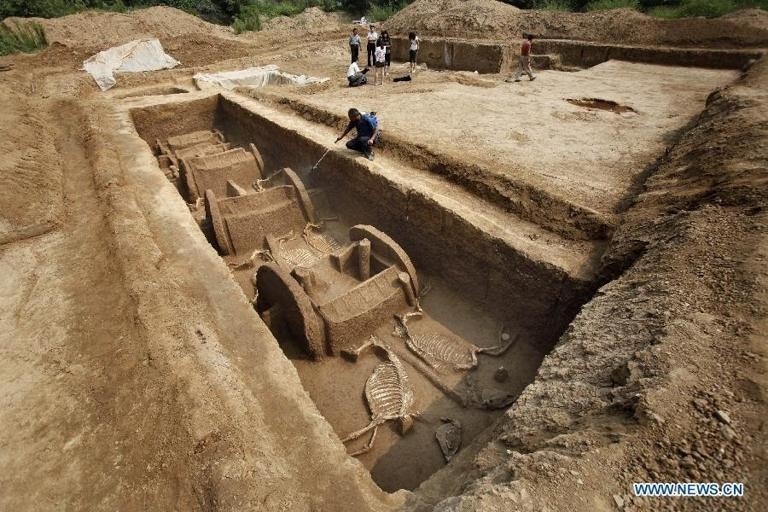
[348,27,362,63]
[513,33,536,82]
[376,28,392,76]
[408,30,420,73]
[334,107,379,162]
[372,38,386,87]
[346,55,367,86]
[365,24,378,67]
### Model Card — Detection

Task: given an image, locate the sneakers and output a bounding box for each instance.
[368,152,375,160]
